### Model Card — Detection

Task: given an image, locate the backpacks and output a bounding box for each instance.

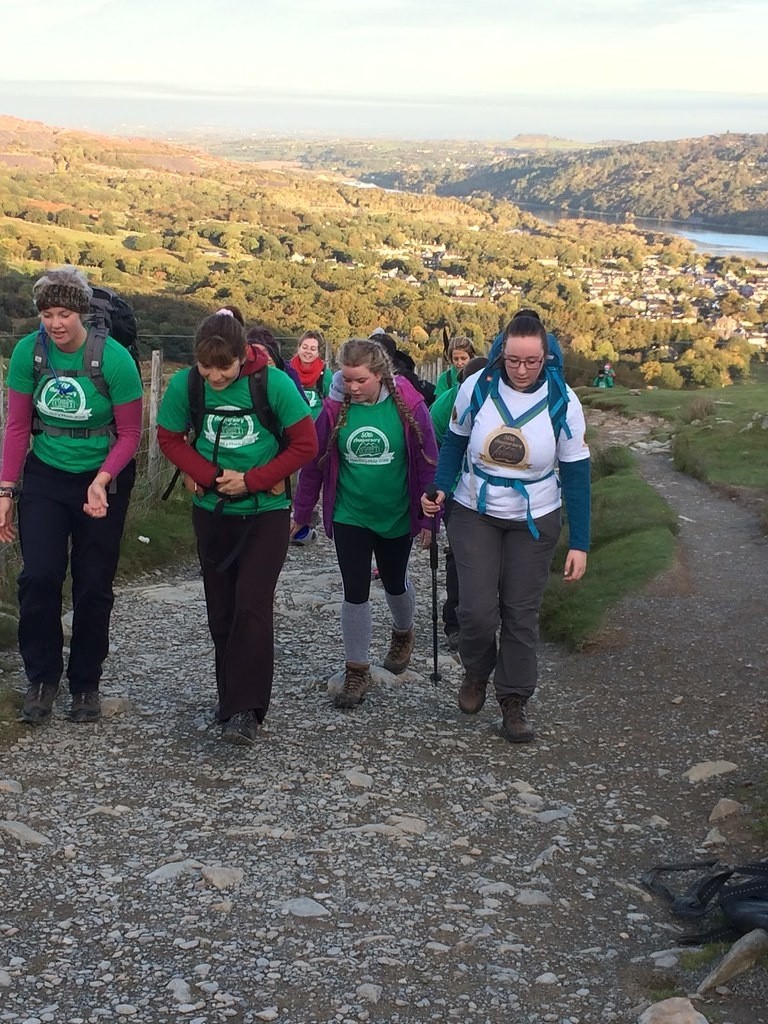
[33,286,144,437]
[466,328,568,488]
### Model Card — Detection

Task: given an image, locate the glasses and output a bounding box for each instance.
[503,354,546,370]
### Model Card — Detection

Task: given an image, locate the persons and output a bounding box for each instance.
[285,329,334,546]
[157,305,320,745]
[429,357,487,653]
[368,333,435,407]
[289,335,441,709]
[436,335,475,402]
[0,265,143,723]
[421,309,592,742]
[593,369,614,390]
[602,363,615,377]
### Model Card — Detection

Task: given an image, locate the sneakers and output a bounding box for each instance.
[459,656,497,713]
[335,661,371,708]
[500,694,534,744]
[22,680,60,723]
[384,621,416,674]
[446,627,459,654]
[216,706,257,746]
[72,690,100,723]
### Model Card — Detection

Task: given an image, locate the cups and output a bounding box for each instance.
[294,525,318,545]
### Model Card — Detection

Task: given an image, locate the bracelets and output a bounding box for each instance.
[0,487,16,497]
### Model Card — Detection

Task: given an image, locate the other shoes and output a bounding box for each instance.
[373,567,379,579]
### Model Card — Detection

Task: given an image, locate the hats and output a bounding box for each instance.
[368,333,398,358]
[457,358,490,384]
[33,266,93,312]
[448,337,476,358]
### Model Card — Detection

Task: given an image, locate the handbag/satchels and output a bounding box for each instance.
[719,862,768,934]
